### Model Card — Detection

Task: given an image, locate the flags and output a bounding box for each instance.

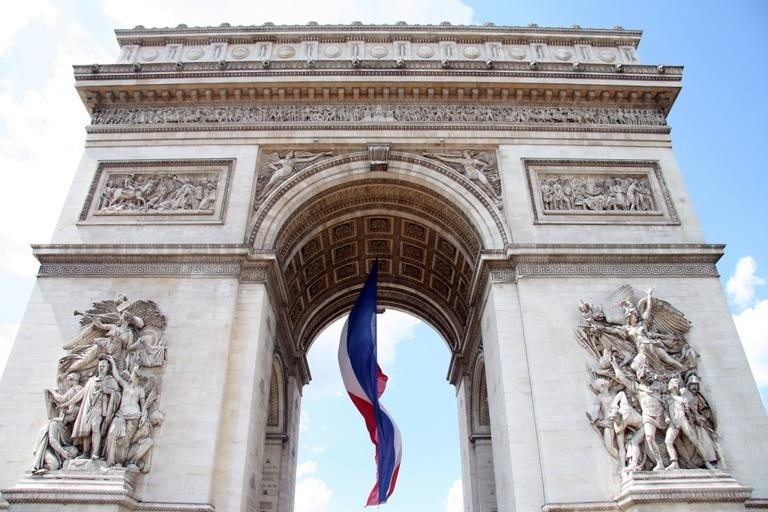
[337,256,402,508]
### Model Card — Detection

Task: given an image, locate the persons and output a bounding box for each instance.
[257,150,324,205]
[31,295,169,475]
[577,287,720,471]
[540,175,656,212]
[432,150,502,204]
[92,108,667,125]
[99,172,218,213]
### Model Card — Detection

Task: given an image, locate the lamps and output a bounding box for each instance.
[367,146,390,173]
